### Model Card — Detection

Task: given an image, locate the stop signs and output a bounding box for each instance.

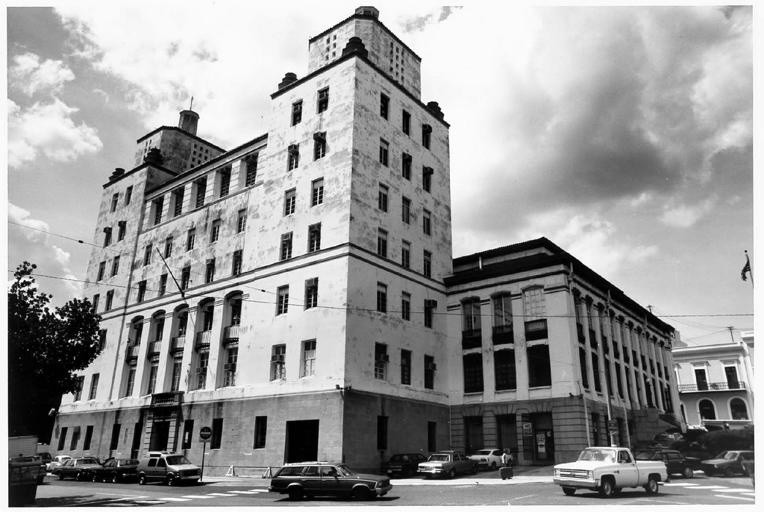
[201,426,212,439]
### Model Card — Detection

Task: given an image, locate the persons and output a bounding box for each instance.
[506,447,513,477]
[500,448,511,480]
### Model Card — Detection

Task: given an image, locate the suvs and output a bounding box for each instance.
[267,460,394,502]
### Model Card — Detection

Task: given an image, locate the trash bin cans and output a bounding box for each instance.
[9,459,42,505]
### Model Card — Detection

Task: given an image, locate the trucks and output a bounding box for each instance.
[9,436,51,507]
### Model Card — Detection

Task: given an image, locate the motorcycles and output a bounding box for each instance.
[499,461,513,480]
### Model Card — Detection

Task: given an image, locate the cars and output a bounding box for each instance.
[698,450,754,479]
[631,446,700,480]
[27,445,202,486]
[385,452,429,479]
[463,447,515,471]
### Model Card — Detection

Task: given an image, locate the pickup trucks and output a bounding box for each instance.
[552,443,669,499]
[415,449,481,479]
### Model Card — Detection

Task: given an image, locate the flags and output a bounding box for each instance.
[740,256,751,282]
